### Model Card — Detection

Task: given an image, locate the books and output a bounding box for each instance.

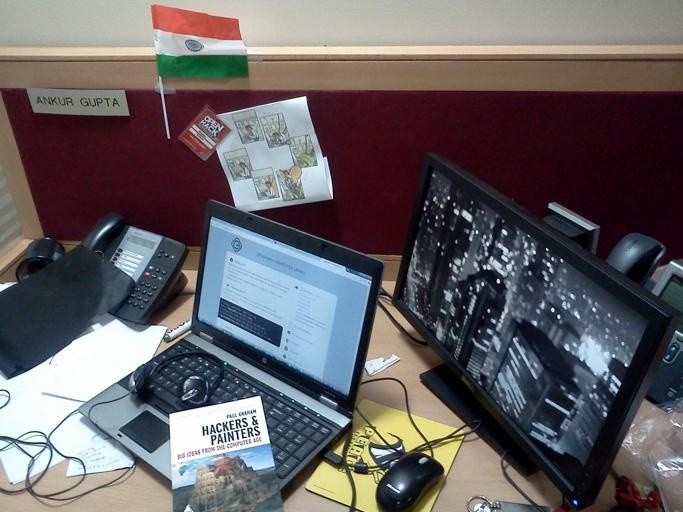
[169,396,285,512]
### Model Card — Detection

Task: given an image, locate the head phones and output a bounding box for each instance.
[128,350,226,407]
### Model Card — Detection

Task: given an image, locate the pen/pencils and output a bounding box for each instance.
[163,318,193,343]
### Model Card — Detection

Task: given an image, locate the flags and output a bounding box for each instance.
[152,4,249,79]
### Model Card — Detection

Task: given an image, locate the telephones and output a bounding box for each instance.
[80,213,189,324]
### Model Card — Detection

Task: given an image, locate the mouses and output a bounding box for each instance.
[376,448,444,512]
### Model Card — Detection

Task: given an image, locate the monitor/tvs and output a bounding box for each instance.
[391,151,681,510]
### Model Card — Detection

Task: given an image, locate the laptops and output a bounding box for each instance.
[77,199,384,498]
